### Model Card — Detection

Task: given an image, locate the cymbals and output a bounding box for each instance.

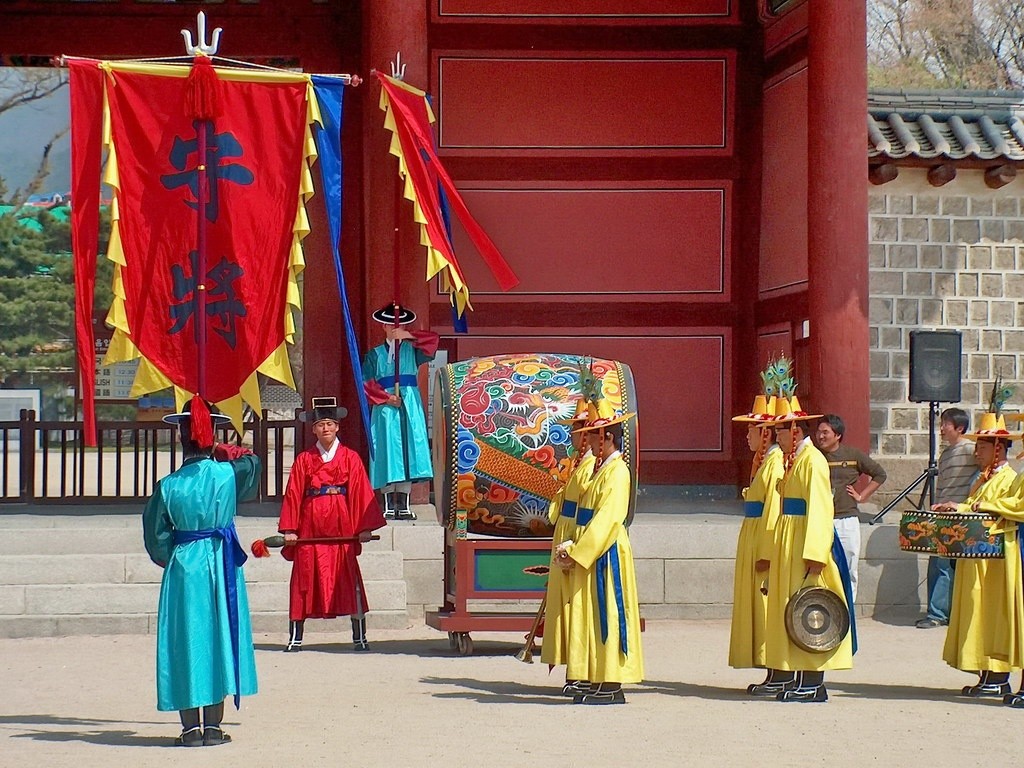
[784,584,851,653]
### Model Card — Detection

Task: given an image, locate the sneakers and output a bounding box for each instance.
[916,618,949,628]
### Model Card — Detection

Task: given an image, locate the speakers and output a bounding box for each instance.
[909,330,962,402]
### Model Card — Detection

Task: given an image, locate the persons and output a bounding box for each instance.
[972,470,1024,707]
[278,397,386,652]
[557,399,643,705]
[930,413,1017,696]
[815,415,888,604]
[142,400,261,746]
[915,408,981,628]
[361,305,439,520]
[541,395,596,698]
[729,395,796,696]
[758,395,852,701]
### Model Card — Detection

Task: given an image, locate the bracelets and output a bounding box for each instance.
[859,496,862,499]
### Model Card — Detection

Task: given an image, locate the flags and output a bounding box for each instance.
[58,52,372,458]
[375,72,520,331]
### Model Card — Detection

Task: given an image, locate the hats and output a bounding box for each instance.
[162,398,233,425]
[570,398,639,434]
[555,398,587,425]
[732,395,777,423]
[372,301,417,326]
[299,397,348,424]
[755,395,824,428]
[966,414,1019,439]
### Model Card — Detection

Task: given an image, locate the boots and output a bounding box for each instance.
[350,618,369,652]
[562,681,592,695]
[748,668,794,695]
[174,701,231,747]
[962,672,1011,697]
[383,493,417,519]
[776,671,829,702]
[284,620,304,653]
[574,682,626,705]
[1003,670,1024,707]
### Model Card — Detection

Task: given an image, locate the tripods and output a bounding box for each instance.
[870,401,941,525]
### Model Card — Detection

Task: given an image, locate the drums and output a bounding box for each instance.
[431,351,639,538]
[899,510,1005,559]
